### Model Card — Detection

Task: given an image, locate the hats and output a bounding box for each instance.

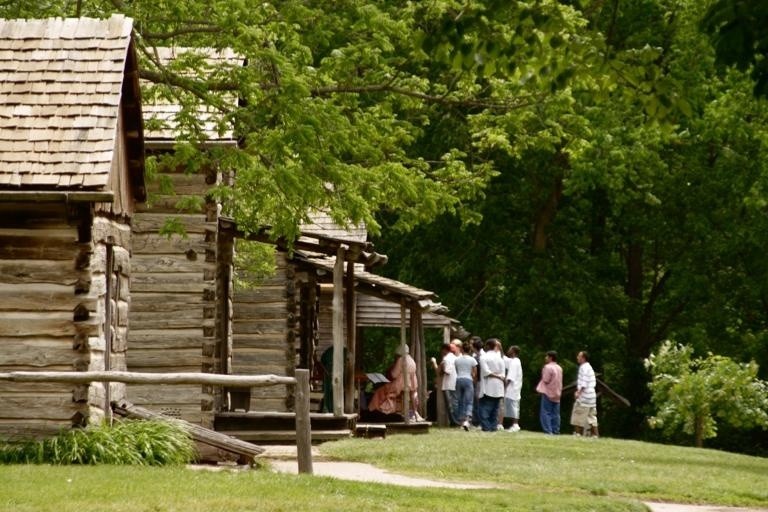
[395,344,409,354]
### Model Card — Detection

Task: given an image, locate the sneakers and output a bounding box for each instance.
[461,421,521,432]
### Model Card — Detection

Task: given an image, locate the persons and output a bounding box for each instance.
[536,350,565,433]
[431,336,524,433]
[319,345,357,411]
[569,352,599,437]
[368,344,426,422]
